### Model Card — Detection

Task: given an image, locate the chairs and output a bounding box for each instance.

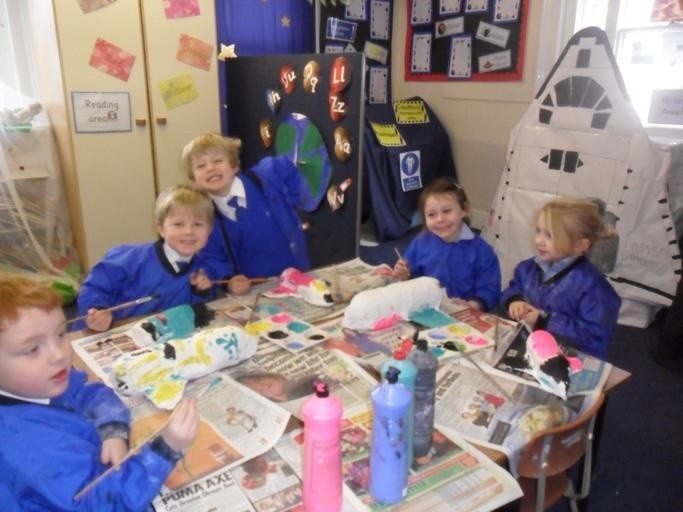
[519,393,607,512]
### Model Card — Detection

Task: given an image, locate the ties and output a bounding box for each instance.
[228,197,247,221]
[176,262,188,270]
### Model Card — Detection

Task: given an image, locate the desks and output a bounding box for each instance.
[69,257,632,512]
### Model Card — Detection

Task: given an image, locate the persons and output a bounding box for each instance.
[500,197,620,367]
[183,132,313,292]
[0,274,200,512]
[393,179,500,312]
[71,187,215,332]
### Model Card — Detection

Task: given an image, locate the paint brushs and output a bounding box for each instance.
[249,292,260,323]
[74,377,222,501]
[394,248,410,277]
[210,278,270,284]
[495,313,499,351]
[67,294,161,324]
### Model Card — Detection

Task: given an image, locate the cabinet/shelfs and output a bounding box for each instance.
[27,0,224,274]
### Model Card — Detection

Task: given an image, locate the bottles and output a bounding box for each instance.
[380,351,417,466]
[370,368,410,505]
[406,339,438,459]
[299,383,342,512]
